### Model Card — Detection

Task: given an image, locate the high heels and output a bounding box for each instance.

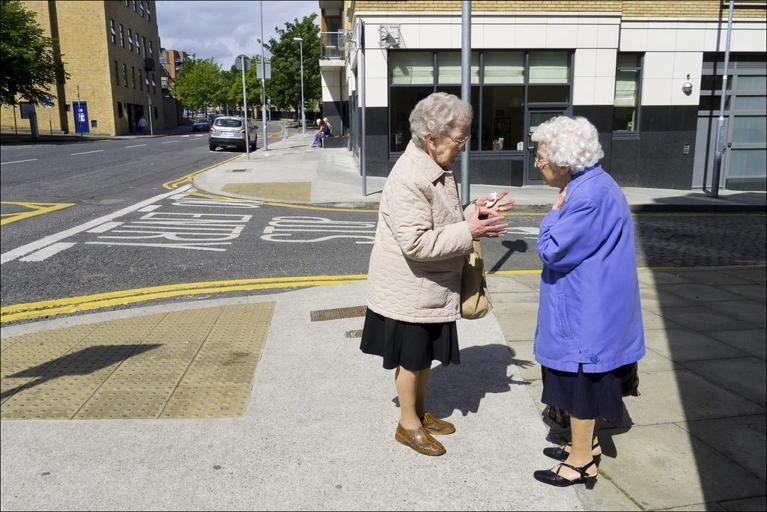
[544,444,601,466]
[533,462,598,490]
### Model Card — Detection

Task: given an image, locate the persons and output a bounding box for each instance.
[322,117,332,135]
[136,115,150,136]
[359,89,515,455]
[525,115,647,488]
[312,119,330,147]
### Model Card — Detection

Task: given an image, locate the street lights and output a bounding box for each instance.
[293,37,306,133]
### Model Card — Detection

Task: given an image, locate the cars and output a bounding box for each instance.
[192,118,212,132]
[208,115,259,151]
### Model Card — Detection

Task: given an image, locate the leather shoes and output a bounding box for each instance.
[394,422,446,456]
[420,411,455,434]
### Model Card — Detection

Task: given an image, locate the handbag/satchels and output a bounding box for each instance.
[460,238,493,320]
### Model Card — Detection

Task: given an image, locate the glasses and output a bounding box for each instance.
[443,132,470,149]
